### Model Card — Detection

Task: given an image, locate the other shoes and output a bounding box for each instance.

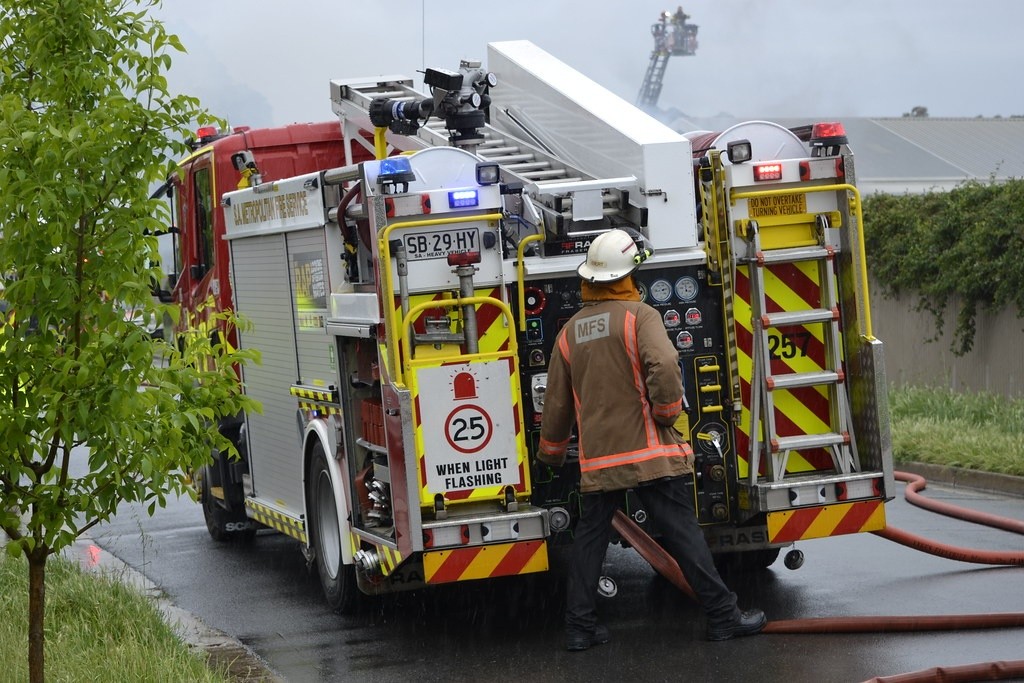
[565,623,612,650]
[705,608,767,641]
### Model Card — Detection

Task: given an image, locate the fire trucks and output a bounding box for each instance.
[146,6,898,625]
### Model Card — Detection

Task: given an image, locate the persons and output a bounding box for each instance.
[535,230,767,650]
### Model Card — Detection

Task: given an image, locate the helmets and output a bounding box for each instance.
[575,228,642,283]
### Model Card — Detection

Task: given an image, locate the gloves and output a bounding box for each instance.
[532,456,558,484]
